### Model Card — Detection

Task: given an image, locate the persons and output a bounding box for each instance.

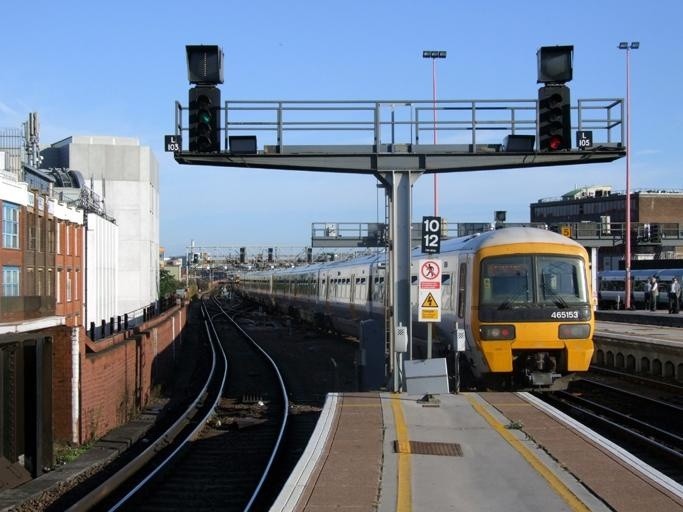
[651,277,658,310]
[643,279,652,310]
[668,278,680,314]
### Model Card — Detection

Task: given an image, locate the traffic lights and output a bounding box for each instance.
[181,42,226,86]
[534,44,576,87]
[186,87,223,154]
[537,84,573,152]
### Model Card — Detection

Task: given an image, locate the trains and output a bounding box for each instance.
[237,223,601,397]
[598,267,683,312]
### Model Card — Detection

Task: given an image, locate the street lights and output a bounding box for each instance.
[618,37,642,311]
[420,49,451,217]
